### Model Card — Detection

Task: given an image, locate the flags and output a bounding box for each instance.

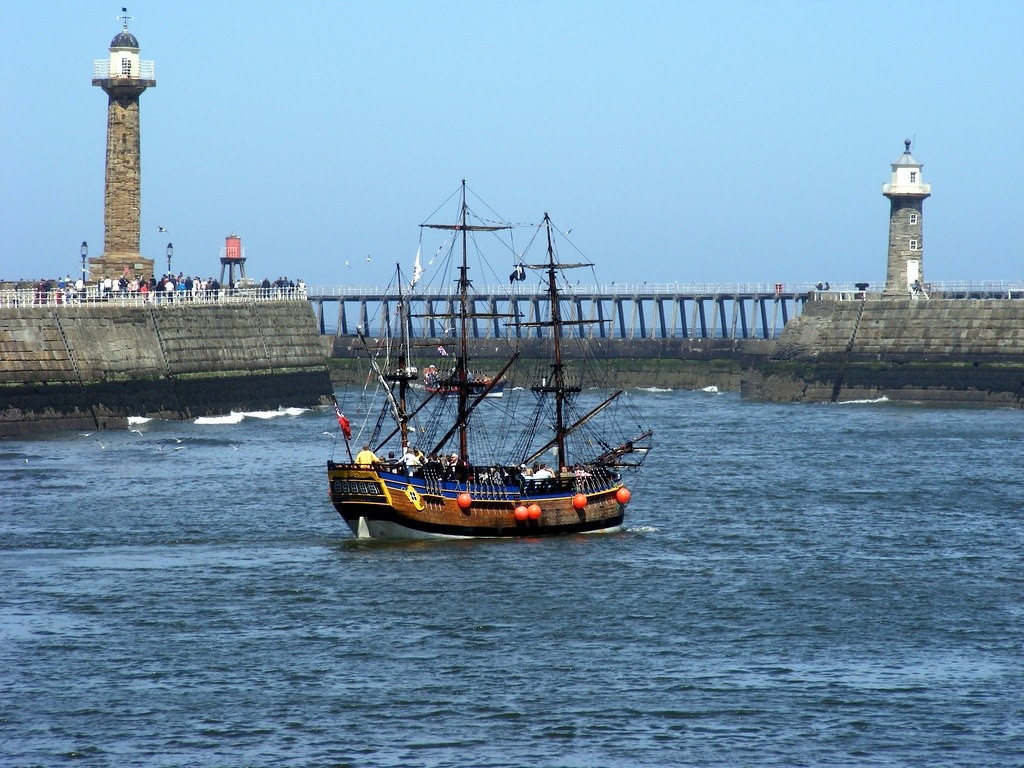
[408,243,423,292]
[335,404,351,441]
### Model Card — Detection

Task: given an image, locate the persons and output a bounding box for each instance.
[12,274,307,309]
[815,281,830,290]
[356,446,593,488]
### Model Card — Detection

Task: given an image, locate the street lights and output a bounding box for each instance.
[80,240,89,301]
[165,242,174,304]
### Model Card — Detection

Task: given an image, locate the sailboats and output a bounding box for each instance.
[326,176,656,539]
